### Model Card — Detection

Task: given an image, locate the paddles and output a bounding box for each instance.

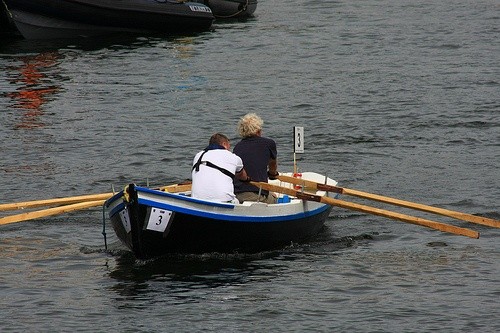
[273,172,500,229]
[1,180,191,213]
[249,180,480,239]
[0,199,106,227]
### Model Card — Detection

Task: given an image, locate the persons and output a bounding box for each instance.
[232,113,281,204]
[191,133,250,205]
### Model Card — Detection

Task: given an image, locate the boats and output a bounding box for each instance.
[1,1,258,47]
[105,172,340,261]
